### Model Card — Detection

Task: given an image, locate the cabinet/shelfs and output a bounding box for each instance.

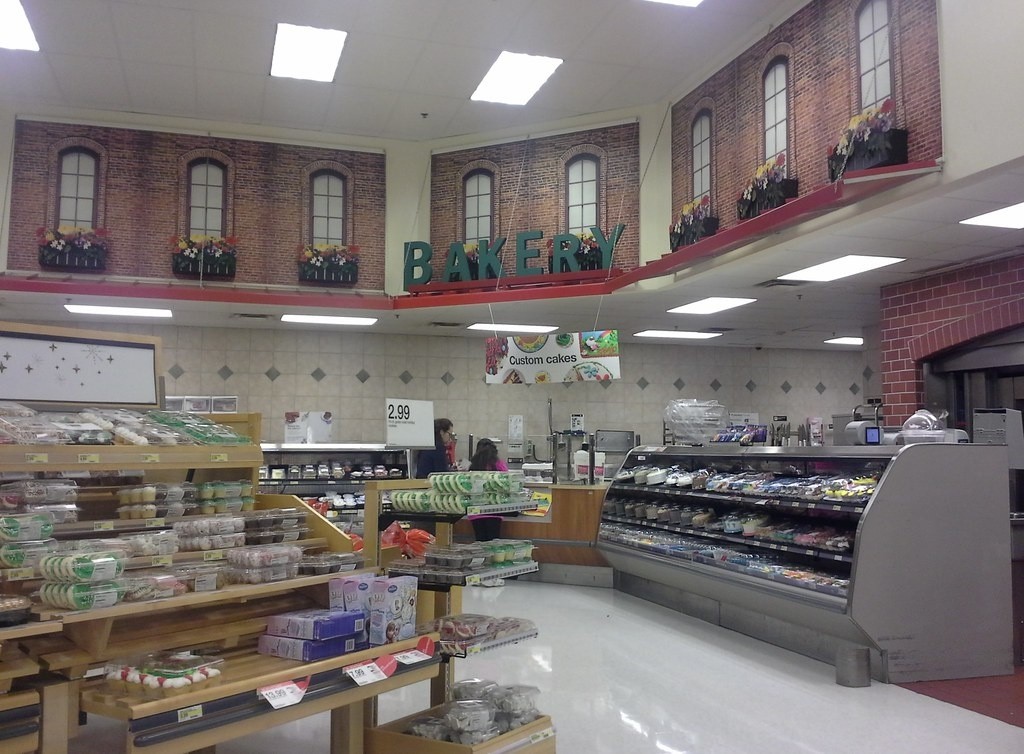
[0,413,557,754]
[596,443,1014,683]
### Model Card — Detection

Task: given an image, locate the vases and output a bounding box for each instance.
[451,263,496,282]
[172,253,236,277]
[830,129,908,183]
[548,247,602,274]
[298,261,358,283]
[38,246,107,270]
[672,218,719,253]
[739,179,798,220]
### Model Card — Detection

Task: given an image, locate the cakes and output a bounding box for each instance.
[486,330,620,383]
[602,466,878,597]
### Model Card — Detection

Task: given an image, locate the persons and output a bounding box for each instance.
[468,438,508,541]
[410,418,453,529]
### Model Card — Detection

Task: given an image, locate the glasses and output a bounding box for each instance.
[445,431,453,438]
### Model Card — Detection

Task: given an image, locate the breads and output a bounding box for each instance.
[389,472,526,512]
[431,614,520,654]
[0,479,363,628]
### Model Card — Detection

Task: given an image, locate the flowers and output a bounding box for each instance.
[669,195,710,249]
[737,154,786,216]
[445,240,495,264]
[547,233,598,256]
[36,225,109,252]
[296,244,360,267]
[827,99,895,170]
[172,234,237,259]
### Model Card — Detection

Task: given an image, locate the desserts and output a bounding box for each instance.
[0,403,250,444]
[105,653,222,700]
[258,464,402,506]
[387,539,534,585]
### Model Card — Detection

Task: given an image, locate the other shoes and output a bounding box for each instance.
[482,578,505,586]
